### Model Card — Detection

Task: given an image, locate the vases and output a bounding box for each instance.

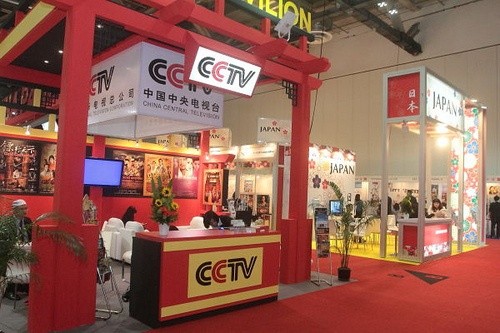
[158,224,170,237]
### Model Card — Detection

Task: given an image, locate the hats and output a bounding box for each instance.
[11,199,26,209]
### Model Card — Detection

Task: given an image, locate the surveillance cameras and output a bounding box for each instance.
[274,12,296,36]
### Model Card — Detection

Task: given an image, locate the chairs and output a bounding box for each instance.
[99,217,204,263]
[328,215,399,251]
[95,231,124,321]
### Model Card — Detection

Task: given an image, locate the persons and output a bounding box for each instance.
[176,157,200,181]
[427,198,448,218]
[376,190,418,218]
[121,206,137,227]
[245,194,268,214]
[2,199,33,301]
[40,155,56,186]
[203,210,233,229]
[489,195,500,240]
[146,158,171,187]
[114,155,143,178]
[354,193,363,220]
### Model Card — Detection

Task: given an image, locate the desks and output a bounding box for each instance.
[129,228,282,330]
[396,218,453,263]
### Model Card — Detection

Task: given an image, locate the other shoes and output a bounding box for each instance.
[7,292,21,301]
[491,236,494,238]
[495,236,499,239]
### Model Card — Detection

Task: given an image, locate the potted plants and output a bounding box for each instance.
[327,180,380,281]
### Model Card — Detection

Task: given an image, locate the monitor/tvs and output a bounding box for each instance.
[329,200,342,215]
[84,157,124,188]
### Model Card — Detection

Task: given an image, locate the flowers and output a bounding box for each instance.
[147,176,180,224]
